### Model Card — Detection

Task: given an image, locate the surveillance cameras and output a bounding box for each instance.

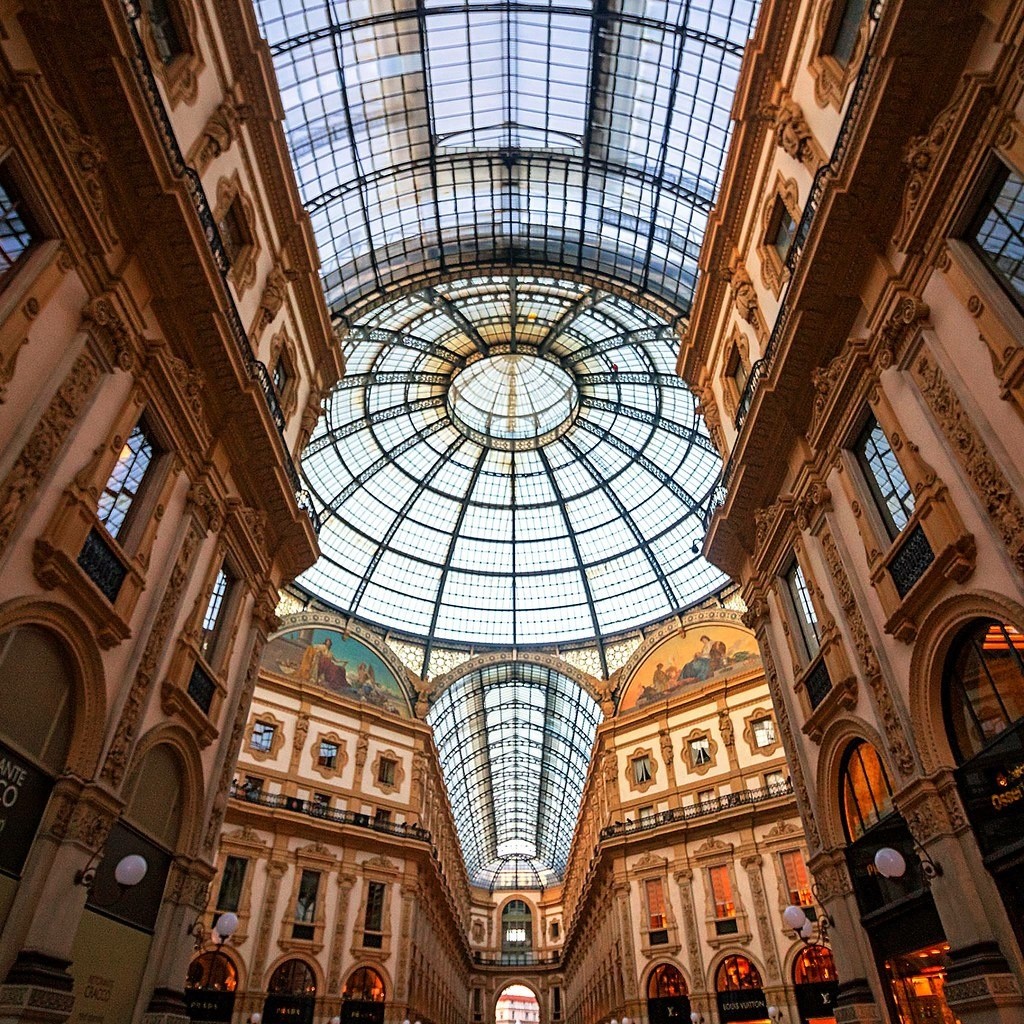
[691,545,699,554]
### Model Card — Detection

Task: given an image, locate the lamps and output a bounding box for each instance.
[74,854,148,909]
[874,835,944,901]
[187,912,239,954]
[690,1013,704,1024]
[783,884,835,953]
[768,1006,783,1024]
[330,1017,340,1024]
[247,1013,260,1024]
[622,1017,635,1024]
